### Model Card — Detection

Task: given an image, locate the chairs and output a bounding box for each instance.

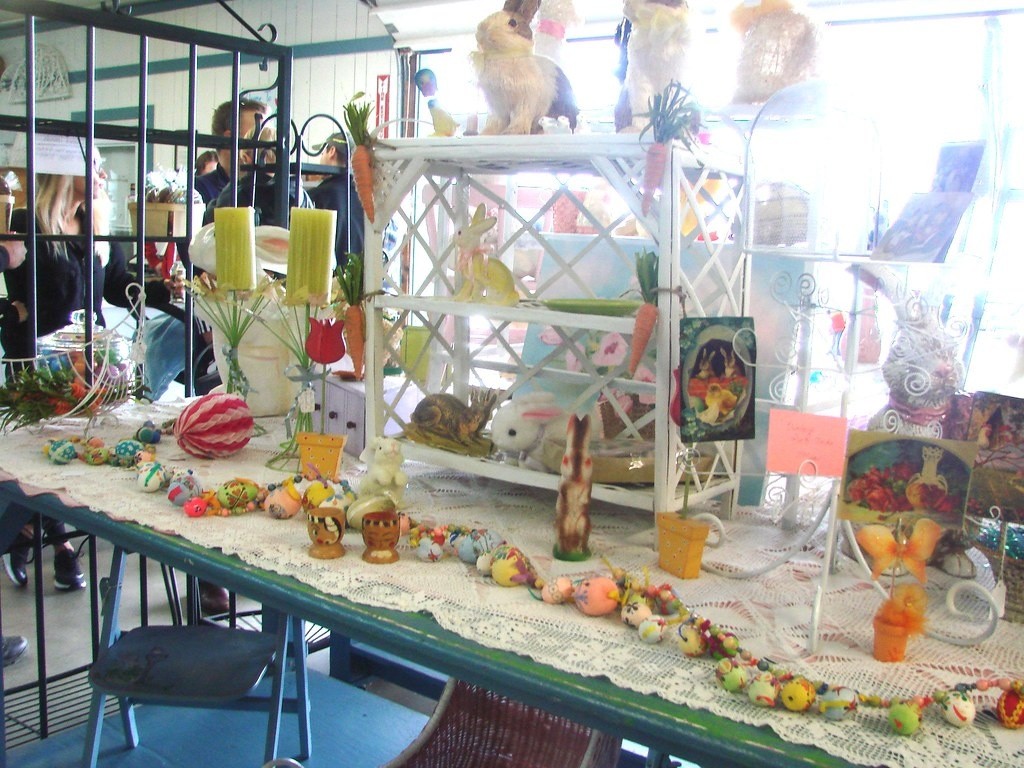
[378,675,622,768]
[82,547,311,768]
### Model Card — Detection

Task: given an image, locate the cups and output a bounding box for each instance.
[306,507,346,559]
[362,511,401,564]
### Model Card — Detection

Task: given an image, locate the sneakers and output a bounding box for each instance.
[3,533,32,587]
[53,548,87,590]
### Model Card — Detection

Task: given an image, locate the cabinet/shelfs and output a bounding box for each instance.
[364,133,755,550]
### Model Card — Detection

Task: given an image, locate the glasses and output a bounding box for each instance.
[92,158,102,173]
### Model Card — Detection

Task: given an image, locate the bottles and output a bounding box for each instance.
[906,447,949,512]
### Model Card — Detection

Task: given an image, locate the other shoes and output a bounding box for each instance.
[2,634,28,668]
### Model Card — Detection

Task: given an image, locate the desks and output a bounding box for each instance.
[1,392,1024,768]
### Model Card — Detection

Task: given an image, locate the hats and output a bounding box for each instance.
[312,132,353,155]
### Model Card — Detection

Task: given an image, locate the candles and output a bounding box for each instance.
[285,206,337,307]
[215,206,256,291]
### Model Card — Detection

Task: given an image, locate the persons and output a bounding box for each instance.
[0,97,397,675]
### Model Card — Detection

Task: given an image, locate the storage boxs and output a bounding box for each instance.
[312,357,417,458]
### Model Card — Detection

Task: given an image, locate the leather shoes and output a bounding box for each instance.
[199,579,230,619]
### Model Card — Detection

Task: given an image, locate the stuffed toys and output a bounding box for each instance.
[867,316,965,441]
[731,0,820,105]
[613,0,692,133]
[491,390,603,473]
[359,436,409,503]
[451,204,520,308]
[411,384,498,449]
